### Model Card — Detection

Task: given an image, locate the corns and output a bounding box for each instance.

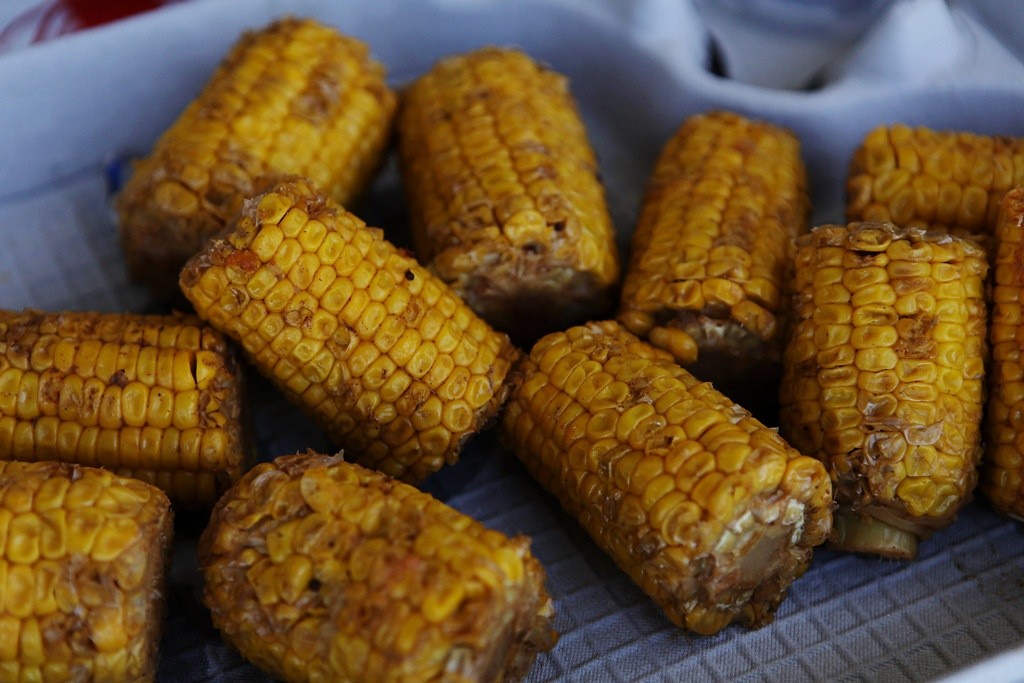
[0,16,1024,683]
